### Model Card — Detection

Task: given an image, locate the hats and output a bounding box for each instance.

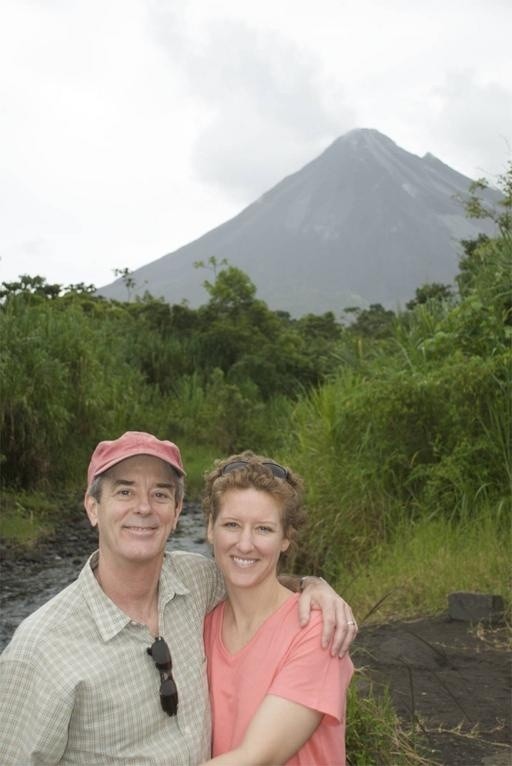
[87,430,187,487]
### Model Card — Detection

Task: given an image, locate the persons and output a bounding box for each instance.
[195,446,361,764]
[0,428,361,764]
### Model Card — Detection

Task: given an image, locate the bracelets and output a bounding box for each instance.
[297,572,330,589]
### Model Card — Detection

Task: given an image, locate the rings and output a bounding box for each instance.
[349,621,356,627]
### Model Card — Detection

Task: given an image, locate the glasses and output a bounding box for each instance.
[146,636,178,716]
[219,460,291,482]
